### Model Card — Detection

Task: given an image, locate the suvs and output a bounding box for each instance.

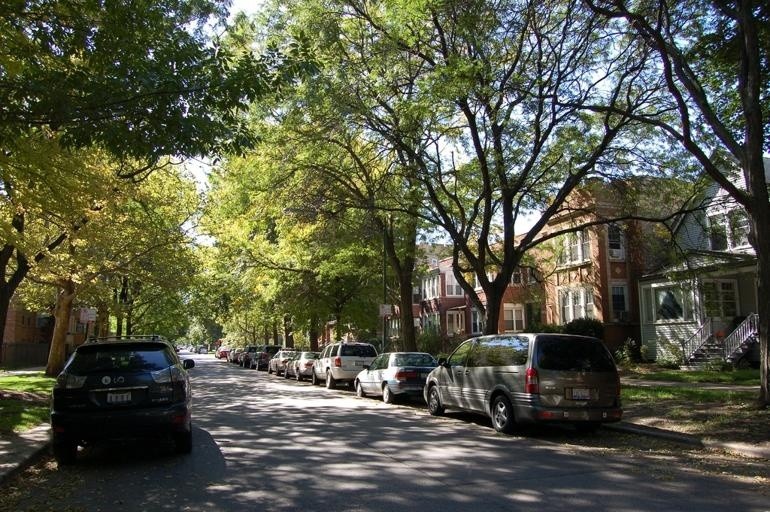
[311,341,378,389]
[51,334,195,459]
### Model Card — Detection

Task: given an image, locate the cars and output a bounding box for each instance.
[215,347,321,381]
[353,350,439,404]
[188,346,208,354]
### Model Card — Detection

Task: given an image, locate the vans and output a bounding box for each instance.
[423,332,623,431]
